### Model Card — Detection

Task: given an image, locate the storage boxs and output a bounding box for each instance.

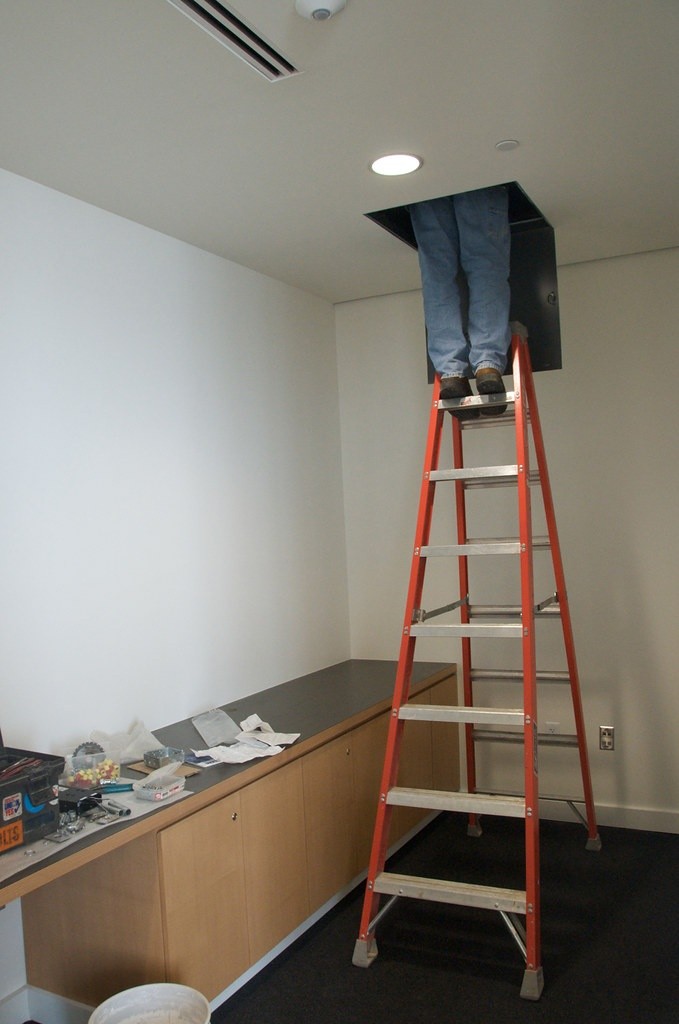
[0,746,66,856]
[143,747,185,769]
[132,776,186,801]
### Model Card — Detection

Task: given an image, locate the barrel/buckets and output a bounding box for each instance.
[89,983,210,1024]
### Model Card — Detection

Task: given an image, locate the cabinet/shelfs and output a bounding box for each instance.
[0,659,457,1024]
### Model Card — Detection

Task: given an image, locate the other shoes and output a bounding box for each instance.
[439,376,479,419]
[476,367,507,415]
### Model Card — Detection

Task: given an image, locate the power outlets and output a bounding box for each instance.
[545,721,560,735]
[599,726,615,750]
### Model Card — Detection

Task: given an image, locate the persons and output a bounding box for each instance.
[404,183,512,421]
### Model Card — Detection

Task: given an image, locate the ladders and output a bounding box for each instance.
[350,319,604,1002]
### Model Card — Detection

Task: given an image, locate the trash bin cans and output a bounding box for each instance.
[87,982,211,1024]
